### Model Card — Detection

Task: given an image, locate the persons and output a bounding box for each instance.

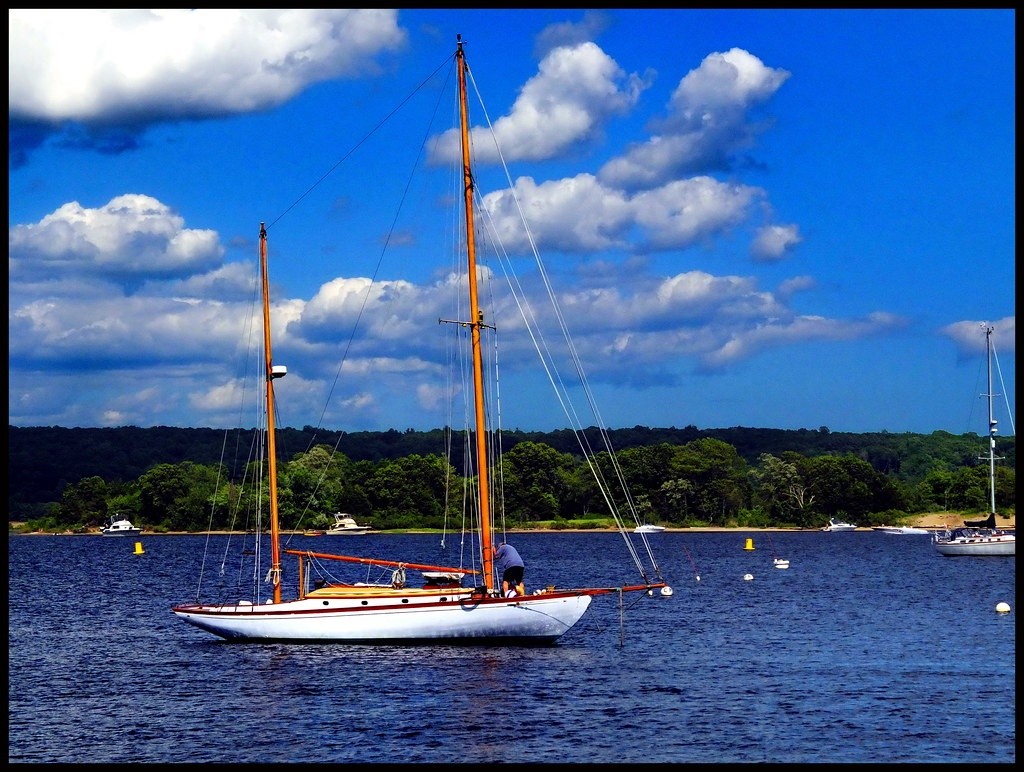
[493,543,524,593]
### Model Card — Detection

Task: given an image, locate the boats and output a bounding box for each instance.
[821,517,861,532]
[94,513,147,541]
[318,506,371,541]
[873,521,927,533]
[633,520,667,536]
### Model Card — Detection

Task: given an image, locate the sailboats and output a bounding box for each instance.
[928,325,1016,556]
[167,31,672,647]
